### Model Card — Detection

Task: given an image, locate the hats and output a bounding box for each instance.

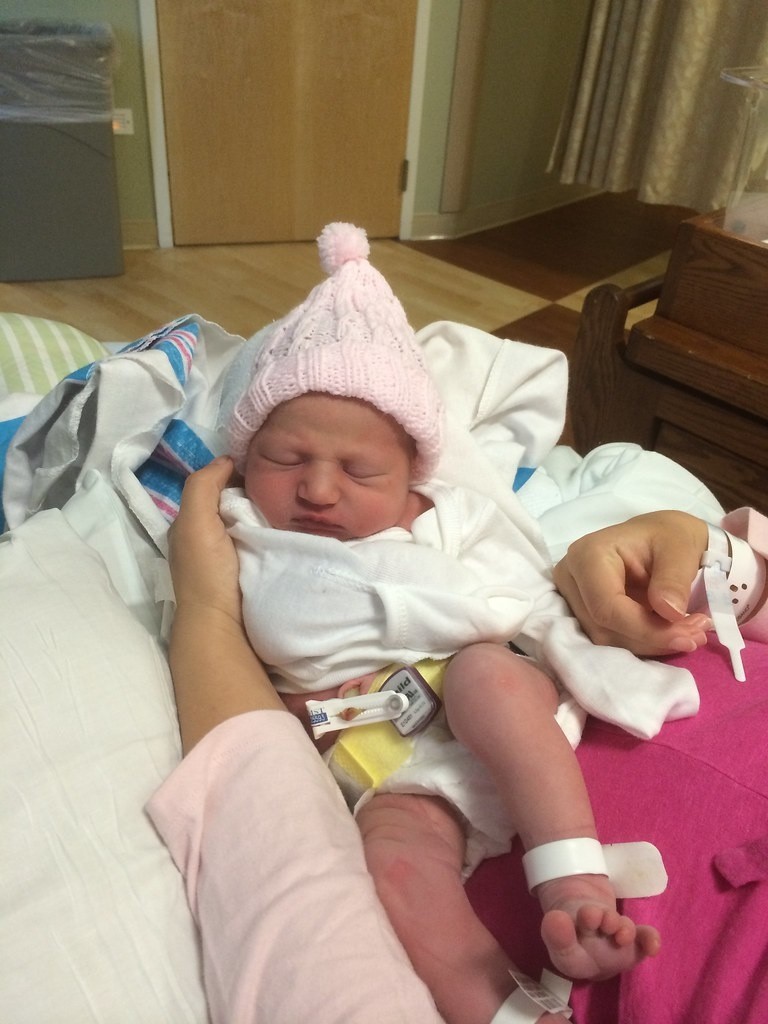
[229,221,443,485]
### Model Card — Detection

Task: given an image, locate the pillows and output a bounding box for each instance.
[0,508,211,1024]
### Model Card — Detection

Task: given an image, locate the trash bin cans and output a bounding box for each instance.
[0,18,126,282]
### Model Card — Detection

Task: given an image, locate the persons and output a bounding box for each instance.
[143,222,768,1024]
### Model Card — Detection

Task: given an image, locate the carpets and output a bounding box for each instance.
[485,306,635,458]
[405,192,700,302]
